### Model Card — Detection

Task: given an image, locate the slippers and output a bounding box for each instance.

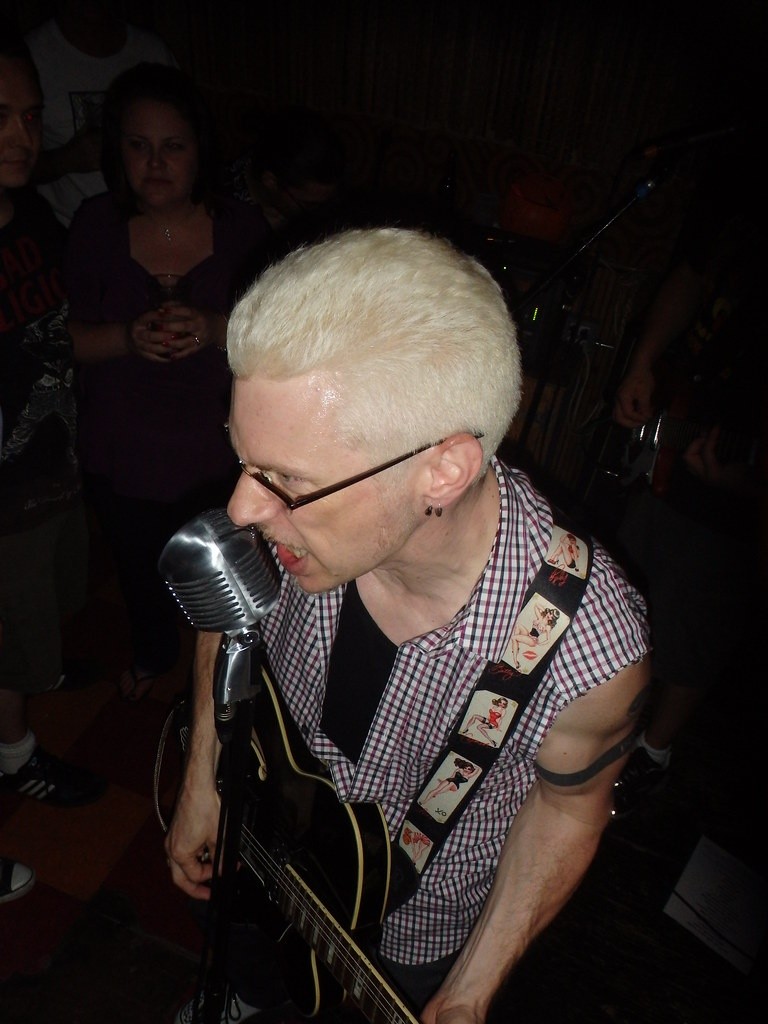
[116,660,160,704]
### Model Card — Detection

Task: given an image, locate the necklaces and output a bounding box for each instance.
[148,209,194,241]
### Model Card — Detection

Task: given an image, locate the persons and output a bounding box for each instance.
[210,106,345,288]
[161,228,650,1024]
[22,0,181,240]
[59,63,267,703]
[0,40,109,809]
[612,163,767,812]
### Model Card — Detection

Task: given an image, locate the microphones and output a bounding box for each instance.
[157,509,282,634]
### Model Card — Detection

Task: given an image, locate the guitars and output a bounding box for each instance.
[150,653,427,1024]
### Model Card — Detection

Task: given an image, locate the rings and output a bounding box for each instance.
[191,335,200,347]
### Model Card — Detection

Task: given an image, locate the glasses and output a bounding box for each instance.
[221,430,484,511]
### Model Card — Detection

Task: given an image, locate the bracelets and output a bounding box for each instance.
[217,310,232,352]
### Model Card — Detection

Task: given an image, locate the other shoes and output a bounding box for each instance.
[174,992,261,1024]
[607,742,673,820]
[0,744,88,807]
[0,857,37,904]
